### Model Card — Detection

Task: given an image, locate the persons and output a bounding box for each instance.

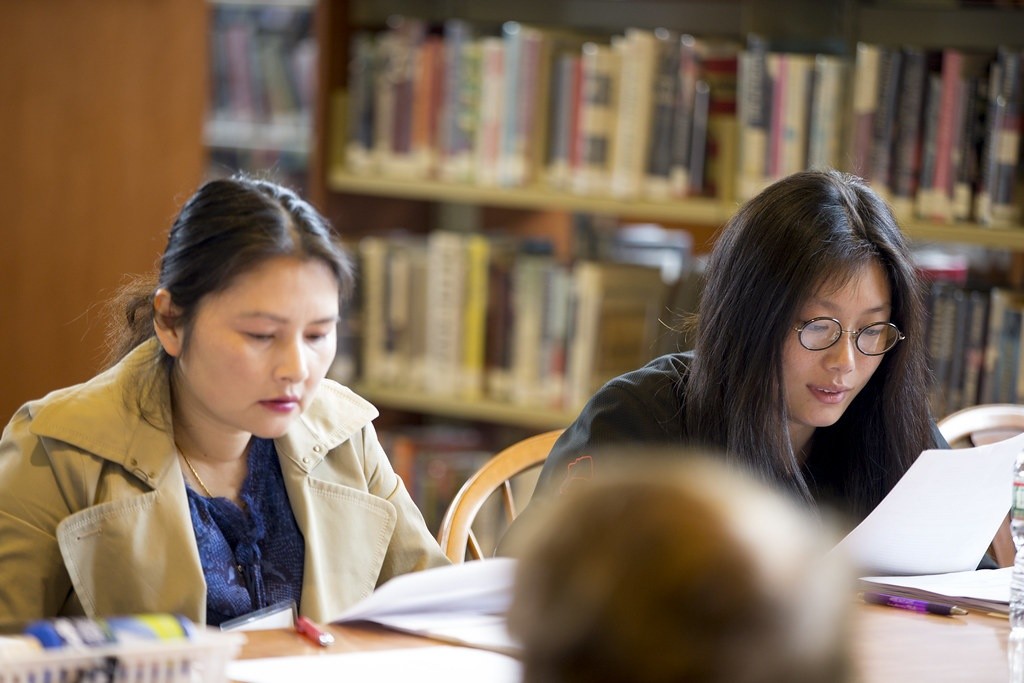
[494,171,996,577]
[503,445,865,683]
[0,177,452,635]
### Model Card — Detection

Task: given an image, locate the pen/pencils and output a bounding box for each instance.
[297,616,334,648]
[857,591,969,616]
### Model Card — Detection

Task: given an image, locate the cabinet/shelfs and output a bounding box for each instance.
[0,0,1024,541]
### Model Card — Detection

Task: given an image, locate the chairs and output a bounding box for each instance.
[929,403,1024,566]
[433,429,568,565]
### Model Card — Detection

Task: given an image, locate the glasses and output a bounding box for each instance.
[791,317,905,356]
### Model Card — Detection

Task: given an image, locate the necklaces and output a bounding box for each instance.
[174,439,215,499]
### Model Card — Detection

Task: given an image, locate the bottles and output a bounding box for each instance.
[1008,434,1024,632]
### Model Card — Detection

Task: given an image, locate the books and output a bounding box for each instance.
[856,566,1013,621]
[334,229,667,416]
[325,13,1024,229]
[906,290,1024,417]
[208,8,311,193]
